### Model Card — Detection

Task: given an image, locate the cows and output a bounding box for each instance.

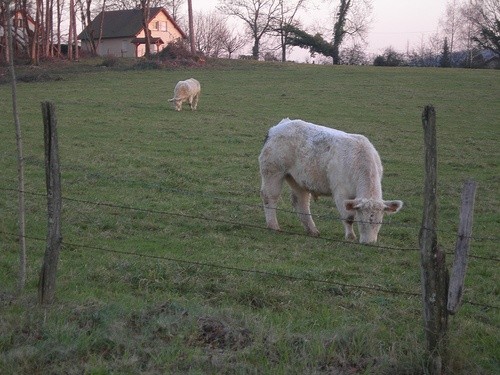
[168,78,201,112]
[258,118,402,245]
